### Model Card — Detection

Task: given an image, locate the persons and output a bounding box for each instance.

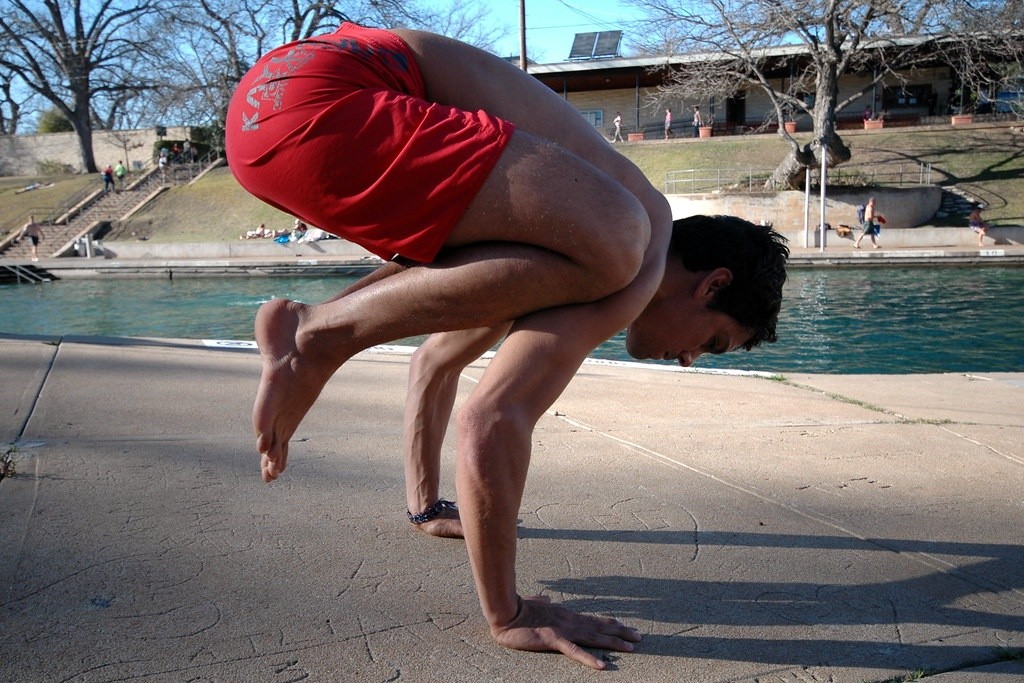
[691,107,701,138]
[927,89,938,116]
[102,165,114,193]
[969,204,989,247]
[183,139,190,162]
[613,112,624,142]
[17,215,45,262]
[226,22,790,671]
[172,144,179,164]
[161,145,170,161]
[114,160,126,182]
[947,87,956,103]
[853,197,880,249]
[294,219,307,231]
[665,108,673,140]
[256,224,265,237]
[159,152,167,184]
[863,104,871,120]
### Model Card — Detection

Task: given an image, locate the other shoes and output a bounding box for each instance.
[853,244,861,248]
[873,246,881,249]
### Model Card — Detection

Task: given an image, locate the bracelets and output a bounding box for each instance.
[407,501,456,524]
